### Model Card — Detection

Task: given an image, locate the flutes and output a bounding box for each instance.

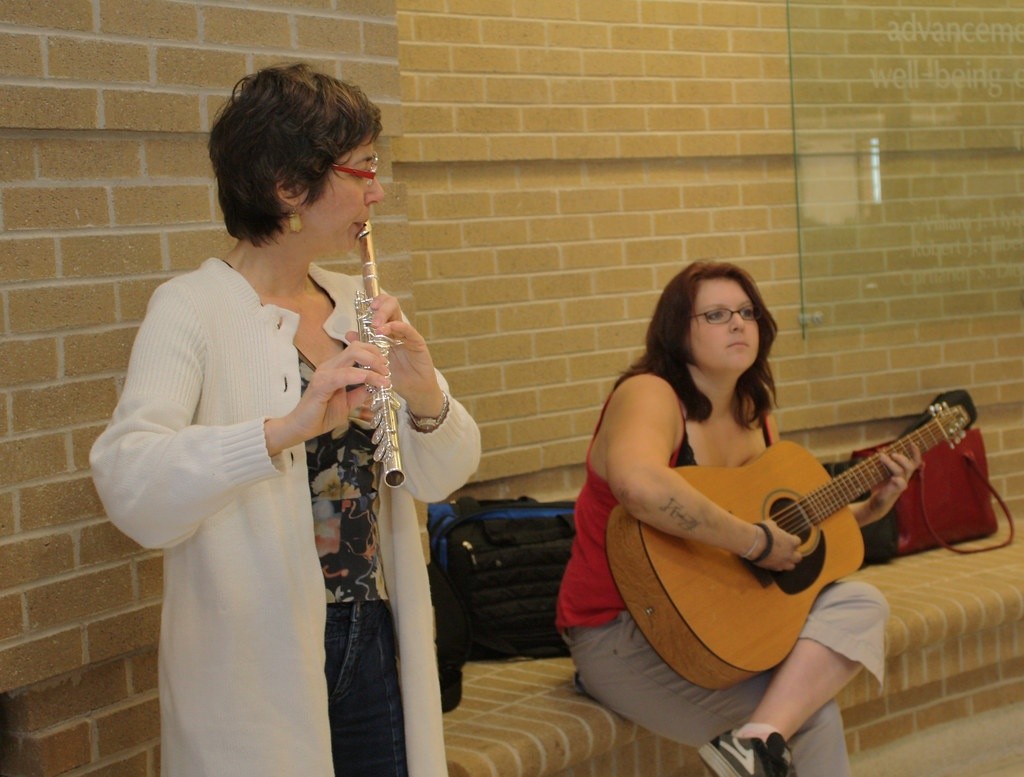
[356,219,406,488]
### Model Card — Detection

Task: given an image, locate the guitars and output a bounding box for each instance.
[604,400,973,691]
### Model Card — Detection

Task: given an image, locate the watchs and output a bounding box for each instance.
[407,392,448,430]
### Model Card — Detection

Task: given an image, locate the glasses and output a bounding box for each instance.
[690,303,763,326]
[327,152,379,186]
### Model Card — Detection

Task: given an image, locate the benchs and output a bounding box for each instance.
[441,516,1024,776]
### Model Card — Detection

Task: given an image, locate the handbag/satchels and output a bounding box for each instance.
[854,428,1016,555]
[424,563,470,714]
[823,458,896,564]
[426,499,581,660]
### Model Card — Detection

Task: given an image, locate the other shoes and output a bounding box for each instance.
[697,728,789,777]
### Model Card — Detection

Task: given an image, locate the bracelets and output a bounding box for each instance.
[740,522,773,564]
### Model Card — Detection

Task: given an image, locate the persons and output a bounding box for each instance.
[556,262,922,777]
[89,66,482,777]
